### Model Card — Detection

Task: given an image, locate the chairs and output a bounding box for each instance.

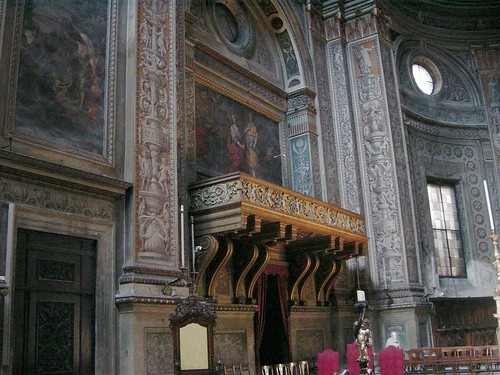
[318,342,404,374]
[403,345,500,375]
[224,361,309,375]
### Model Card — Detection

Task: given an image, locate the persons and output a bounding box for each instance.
[354,319,373,361]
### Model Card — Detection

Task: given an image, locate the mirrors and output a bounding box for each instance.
[168,295,218,375]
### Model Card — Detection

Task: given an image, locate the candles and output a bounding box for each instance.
[382,258,387,288]
[357,290,365,301]
[483,180,494,234]
[431,257,435,287]
[180,213,184,267]
[191,224,195,272]
